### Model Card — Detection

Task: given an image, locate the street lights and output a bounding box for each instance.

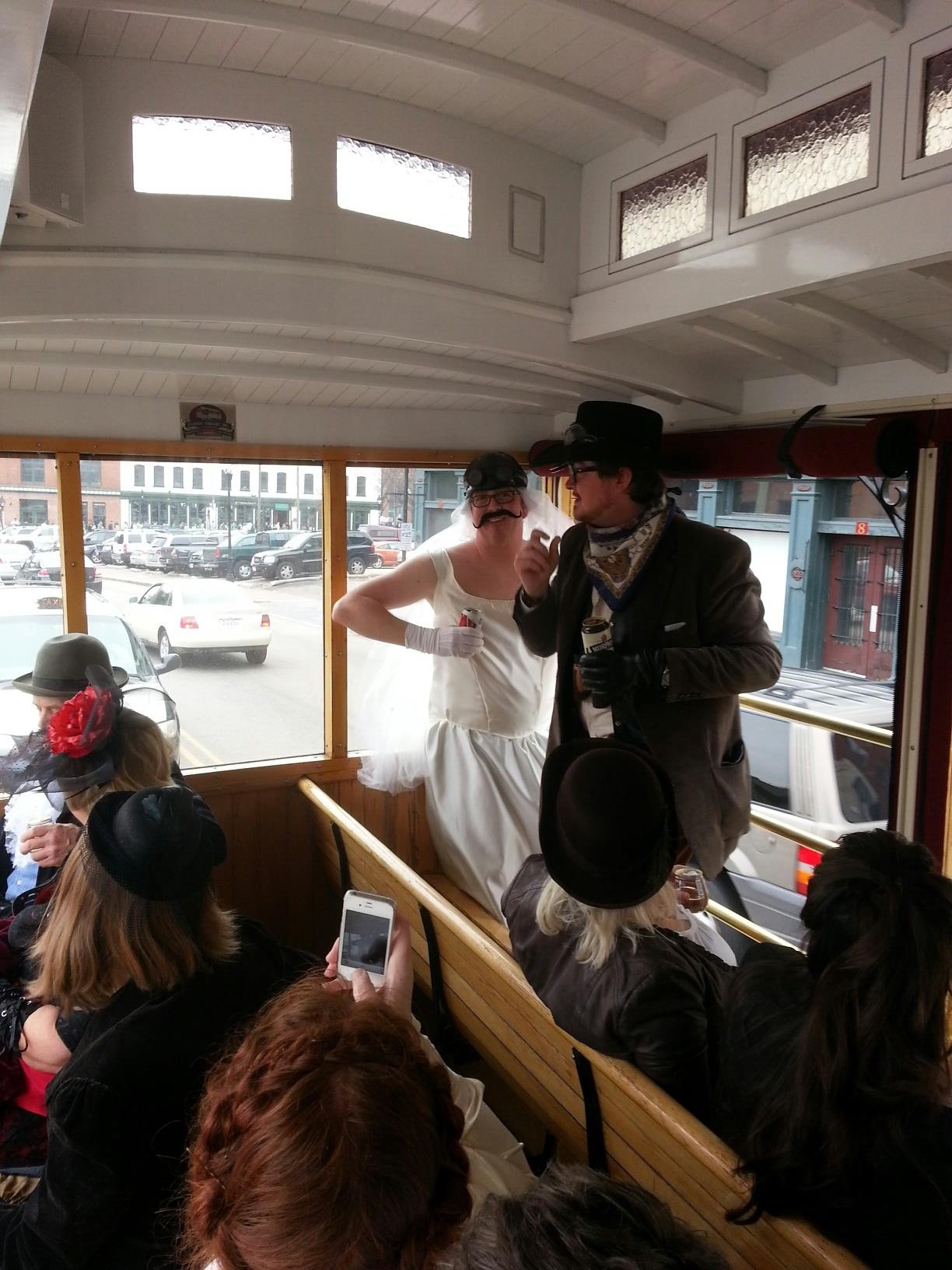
[221,463,233,582]
[377,492,408,562]
[0,497,5,525]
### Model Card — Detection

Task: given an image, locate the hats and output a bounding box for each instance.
[537,739,676,908]
[528,400,663,477]
[82,785,228,904]
[14,632,129,698]
[464,453,528,486]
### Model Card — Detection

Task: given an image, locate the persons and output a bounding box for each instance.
[0,786,314,1268]
[186,917,537,1270]
[180,522,185,529]
[232,522,240,529]
[220,522,227,530]
[11,517,47,526]
[0,684,180,1170]
[265,521,289,531]
[514,398,783,884]
[718,829,952,1269]
[501,737,741,1114]
[133,520,158,529]
[460,1164,730,1270]
[87,520,128,532]
[332,455,576,929]
[0,633,187,977]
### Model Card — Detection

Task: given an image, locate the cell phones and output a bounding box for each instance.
[336,889,398,988]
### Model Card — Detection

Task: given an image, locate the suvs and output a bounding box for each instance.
[0,580,182,768]
[251,530,378,580]
[357,524,401,541]
[217,530,303,580]
[702,666,896,953]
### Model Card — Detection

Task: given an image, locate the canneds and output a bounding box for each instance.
[460,607,482,630]
[581,617,614,653]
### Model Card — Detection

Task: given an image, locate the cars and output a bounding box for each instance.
[146,529,255,577]
[372,541,415,569]
[120,579,273,664]
[0,525,59,580]
[13,550,103,594]
[83,530,167,571]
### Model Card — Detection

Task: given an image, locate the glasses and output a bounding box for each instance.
[472,490,518,507]
[568,463,597,483]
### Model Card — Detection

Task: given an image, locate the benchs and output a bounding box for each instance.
[299,778,874,1270]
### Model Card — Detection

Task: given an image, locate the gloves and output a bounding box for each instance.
[405,623,485,658]
[579,649,649,709]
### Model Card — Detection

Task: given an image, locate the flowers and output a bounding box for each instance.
[45,685,116,759]
